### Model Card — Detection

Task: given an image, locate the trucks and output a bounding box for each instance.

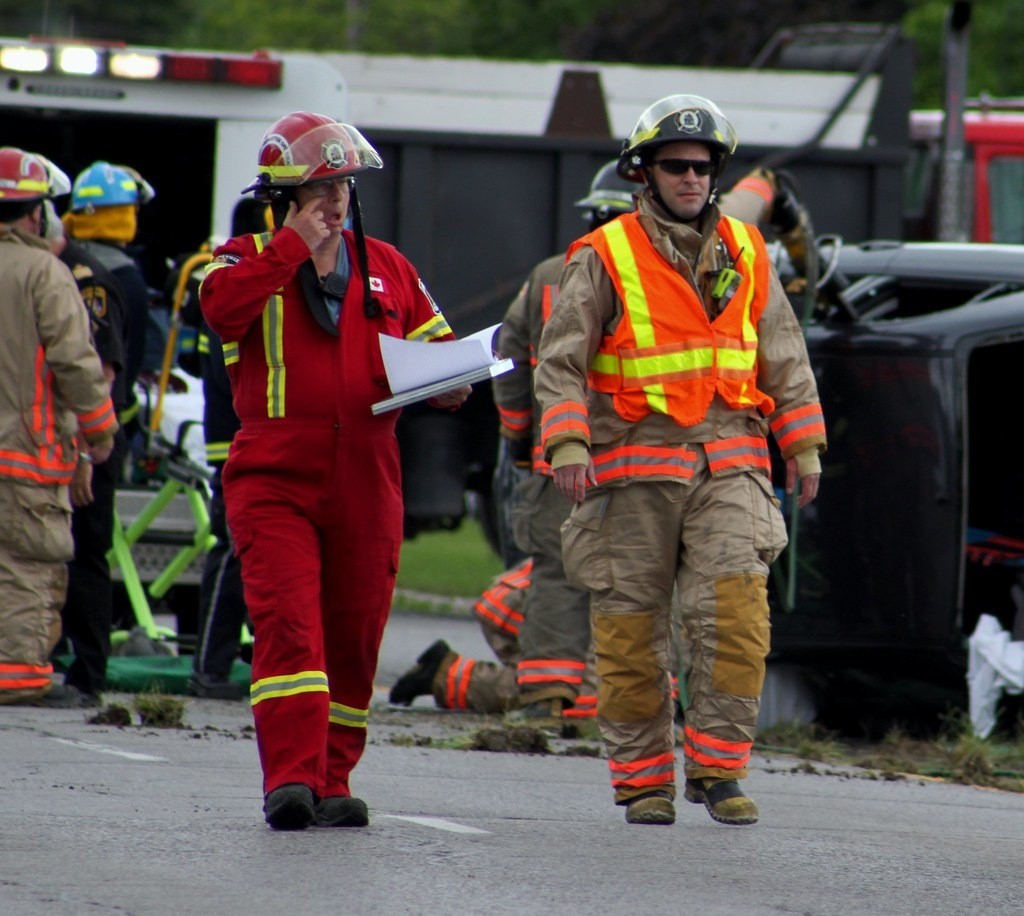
[1,1,1022,560]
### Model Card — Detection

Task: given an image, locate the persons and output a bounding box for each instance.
[533,95,828,822]
[390,156,780,734]
[190,197,269,698]
[0,146,156,709]
[198,114,474,829]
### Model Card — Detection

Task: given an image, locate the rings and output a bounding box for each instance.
[75,488,82,498]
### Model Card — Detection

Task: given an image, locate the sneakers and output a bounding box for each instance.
[314,796,369,828]
[683,775,760,823]
[264,784,316,828]
[0,677,81,708]
[626,789,675,825]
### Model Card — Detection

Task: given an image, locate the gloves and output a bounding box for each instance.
[771,188,800,236]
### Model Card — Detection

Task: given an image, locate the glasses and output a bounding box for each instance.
[298,174,357,196]
[650,159,715,176]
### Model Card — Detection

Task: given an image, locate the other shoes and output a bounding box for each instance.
[526,698,566,738]
[185,671,243,702]
[81,691,102,707]
[389,640,450,704]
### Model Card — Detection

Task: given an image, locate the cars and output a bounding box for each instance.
[776,240,1022,733]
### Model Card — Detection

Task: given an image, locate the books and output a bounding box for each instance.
[371,323,514,415]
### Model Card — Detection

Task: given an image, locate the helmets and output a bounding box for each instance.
[241,111,371,197]
[70,161,142,211]
[0,146,54,202]
[615,103,740,182]
[572,158,647,219]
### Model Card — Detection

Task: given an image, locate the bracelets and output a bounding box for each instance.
[76,452,92,462]
